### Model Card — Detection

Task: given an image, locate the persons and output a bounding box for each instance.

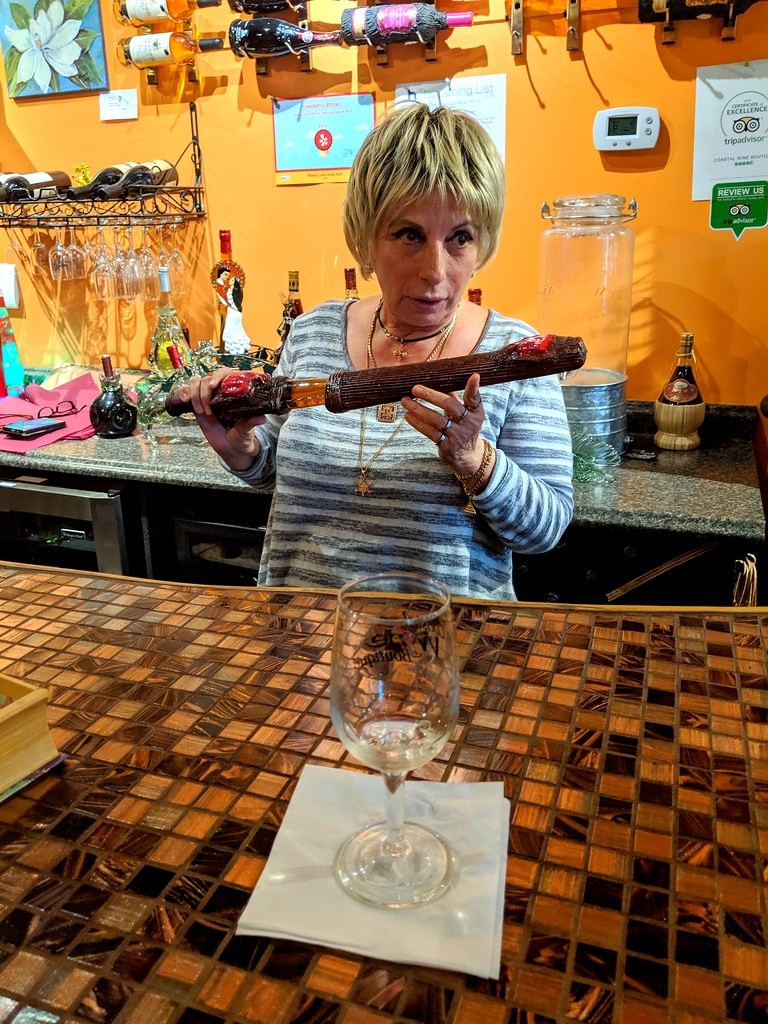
[215,267,250,354]
[180,97,575,605]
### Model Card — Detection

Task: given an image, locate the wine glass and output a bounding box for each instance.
[330,573,459,910]
[2,212,186,304]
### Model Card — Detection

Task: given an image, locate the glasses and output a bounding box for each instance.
[37,401,87,419]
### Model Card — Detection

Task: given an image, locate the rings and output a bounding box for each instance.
[463,395,483,409]
[435,434,446,447]
[453,404,468,421]
[440,417,452,432]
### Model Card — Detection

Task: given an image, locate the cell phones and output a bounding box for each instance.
[4,418,66,434]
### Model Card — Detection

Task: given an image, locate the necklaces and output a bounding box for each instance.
[354,298,461,496]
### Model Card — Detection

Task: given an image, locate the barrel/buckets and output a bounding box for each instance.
[561,367,632,461]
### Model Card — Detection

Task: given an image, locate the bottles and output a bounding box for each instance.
[113,0,222,27]
[68,162,140,200]
[229,19,344,59]
[654,332,705,450]
[341,3,473,47]
[227,0,311,15]
[148,266,197,420]
[209,229,252,355]
[116,32,224,68]
[89,354,138,438]
[638,0,763,22]
[276,271,304,339]
[468,288,482,306]
[542,194,639,385]
[96,159,179,200]
[343,268,360,300]
[0,170,72,202]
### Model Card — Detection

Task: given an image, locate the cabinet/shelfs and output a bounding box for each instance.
[134,479,768,610]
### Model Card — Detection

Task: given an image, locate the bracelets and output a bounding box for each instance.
[455,438,492,515]
